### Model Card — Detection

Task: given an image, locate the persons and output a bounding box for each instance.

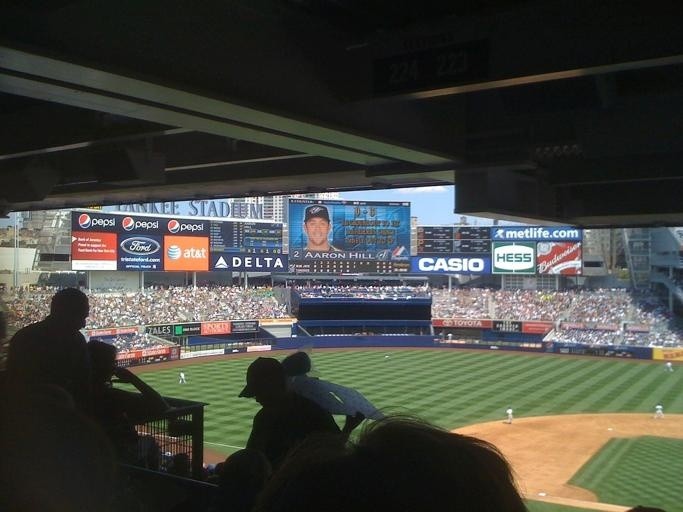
[655,404,665,418]
[666,362,673,372]
[0,287,172,511]
[302,205,339,252]
[177,350,528,509]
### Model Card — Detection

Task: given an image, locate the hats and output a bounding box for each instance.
[238,356,282,400]
[303,205,330,223]
